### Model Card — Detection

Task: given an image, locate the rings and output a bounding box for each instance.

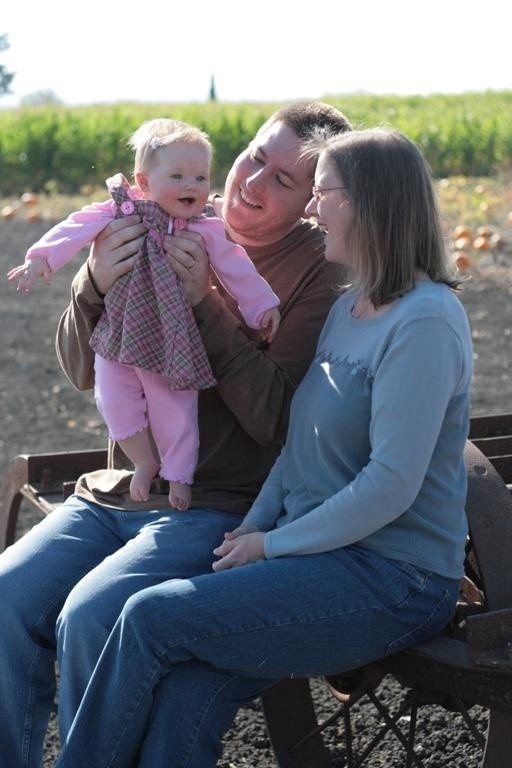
[188,261,199,270]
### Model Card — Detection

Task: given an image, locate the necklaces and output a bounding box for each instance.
[350,268,424,319]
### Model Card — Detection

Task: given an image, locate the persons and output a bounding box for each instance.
[0,101,351,768]
[7,118,282,512]
[52,129,471,768]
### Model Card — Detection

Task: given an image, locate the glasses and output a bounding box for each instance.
[312,185,353,201]
